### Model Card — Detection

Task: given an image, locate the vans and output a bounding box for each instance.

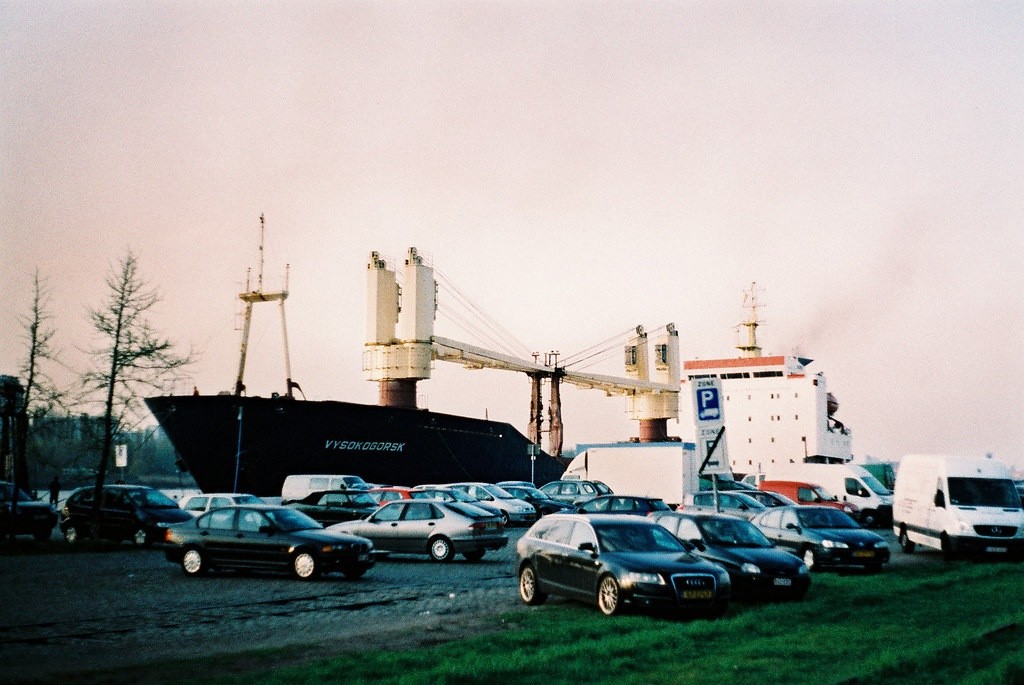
[756,480,859,521]
[760,462,893,529]
[281,474,371,503]
[892,452,1024,562]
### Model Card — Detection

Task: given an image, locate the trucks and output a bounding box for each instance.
[560,442,699,509]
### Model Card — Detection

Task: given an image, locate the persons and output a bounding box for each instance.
[50,476,61,509]
[112,480,125,495]
[194,387,198,396]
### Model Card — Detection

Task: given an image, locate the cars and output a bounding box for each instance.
[282,489,383,528]
[60,484,195,550]
[358,479,623,528]
[551,494,674,517]
[645,510,812,604]
[675,472,801,522]
[1012,477,1024,503]
[515,513,731,621]
[178,492,267,519]
[163,503,376,581]
[0,481,59,543]
[325,498,509,564]
